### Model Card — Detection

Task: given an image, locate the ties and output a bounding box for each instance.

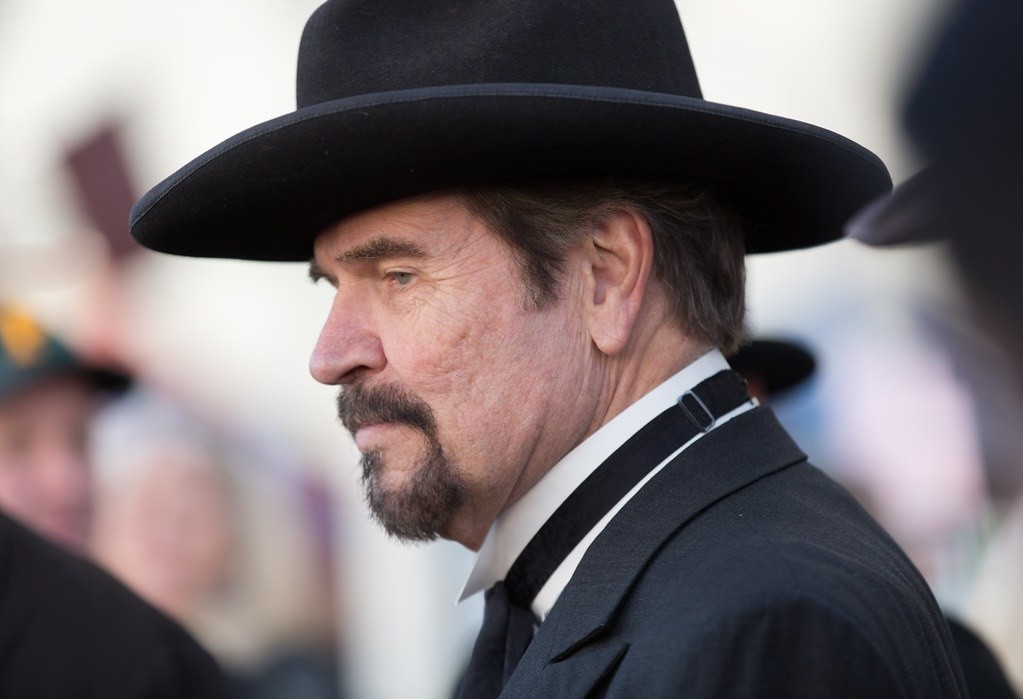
[454,368,751,699]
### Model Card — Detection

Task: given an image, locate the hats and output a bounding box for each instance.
[130,0,894,262]
[849,0,1023,244]
[0,296,130,395]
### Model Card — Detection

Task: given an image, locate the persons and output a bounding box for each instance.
[127,0,1022,699]
[0,303,341,699]
